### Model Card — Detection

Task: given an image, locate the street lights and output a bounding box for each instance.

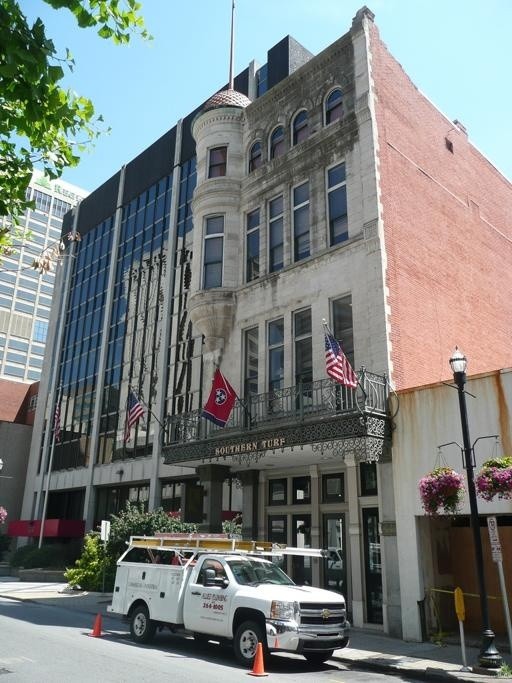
[449,343,503,668]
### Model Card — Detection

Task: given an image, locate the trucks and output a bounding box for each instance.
[107,532,351,663]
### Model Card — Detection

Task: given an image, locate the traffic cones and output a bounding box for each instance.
[248,642,269,677]
[88,612,104,637]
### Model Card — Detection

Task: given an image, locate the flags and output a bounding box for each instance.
[201,368,237,428]
[124,388,144,443]
[325,331,357,390]
[54,404,61,442]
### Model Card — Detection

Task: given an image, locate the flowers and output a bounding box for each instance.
[474,455,512,502]
[418,463,465,521]
